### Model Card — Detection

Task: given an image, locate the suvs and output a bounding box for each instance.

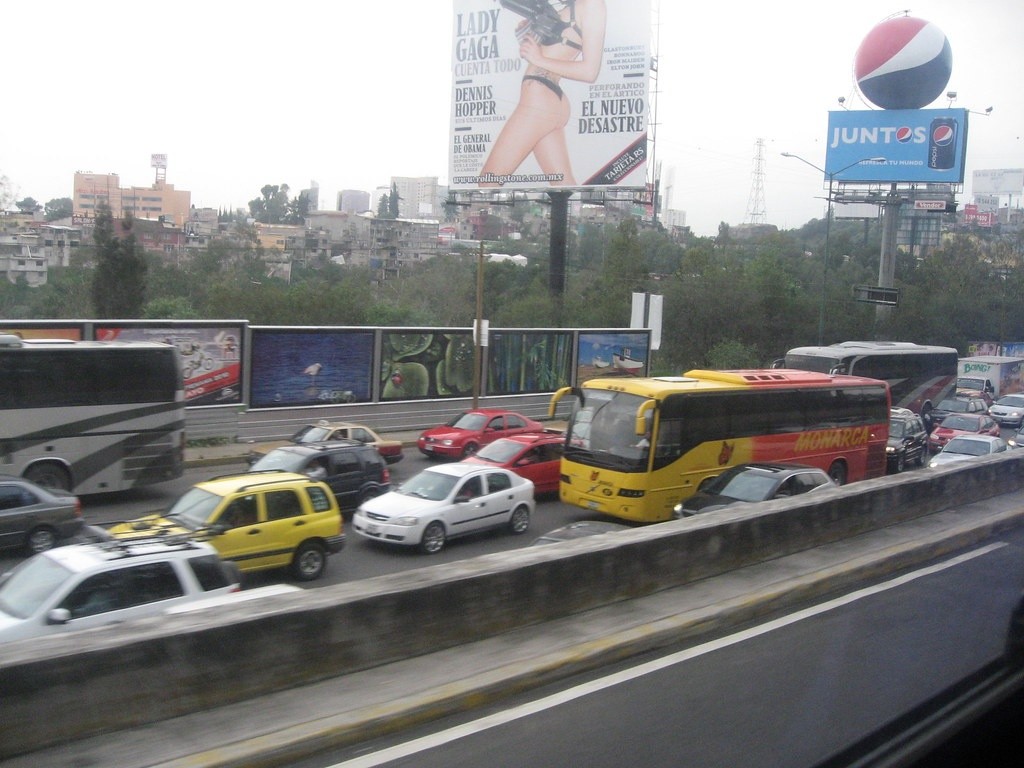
[100,469,347,586]
[240,437,391,515]
[887,406,928,474]
[0,510,245,645]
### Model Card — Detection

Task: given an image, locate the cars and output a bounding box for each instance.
[671,460,841,521]
[351,463,537,556]
[417,406,544,462]
[927,413,1001,457]
[923,395,990,435]
[988,392,1024,428]
[456,427,591,502]
[926,434,1012,468]
[1006,423,1024,451]
[1,473,87,556]
[245,419,404,467]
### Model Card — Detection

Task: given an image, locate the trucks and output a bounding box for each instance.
[957,355,1024,402]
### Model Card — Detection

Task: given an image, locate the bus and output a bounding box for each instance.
[770,339,959,431]
[547,368,892,525]
[0,334,187,501]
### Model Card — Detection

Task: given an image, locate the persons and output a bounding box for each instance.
[458,484,473,497]
[338,434,346,438]
[230,503,252,528]
[305,459,327,479]
[477,0,607,188]
[631,430,661,453]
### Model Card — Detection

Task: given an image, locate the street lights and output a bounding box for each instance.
[780,151,887,345]
